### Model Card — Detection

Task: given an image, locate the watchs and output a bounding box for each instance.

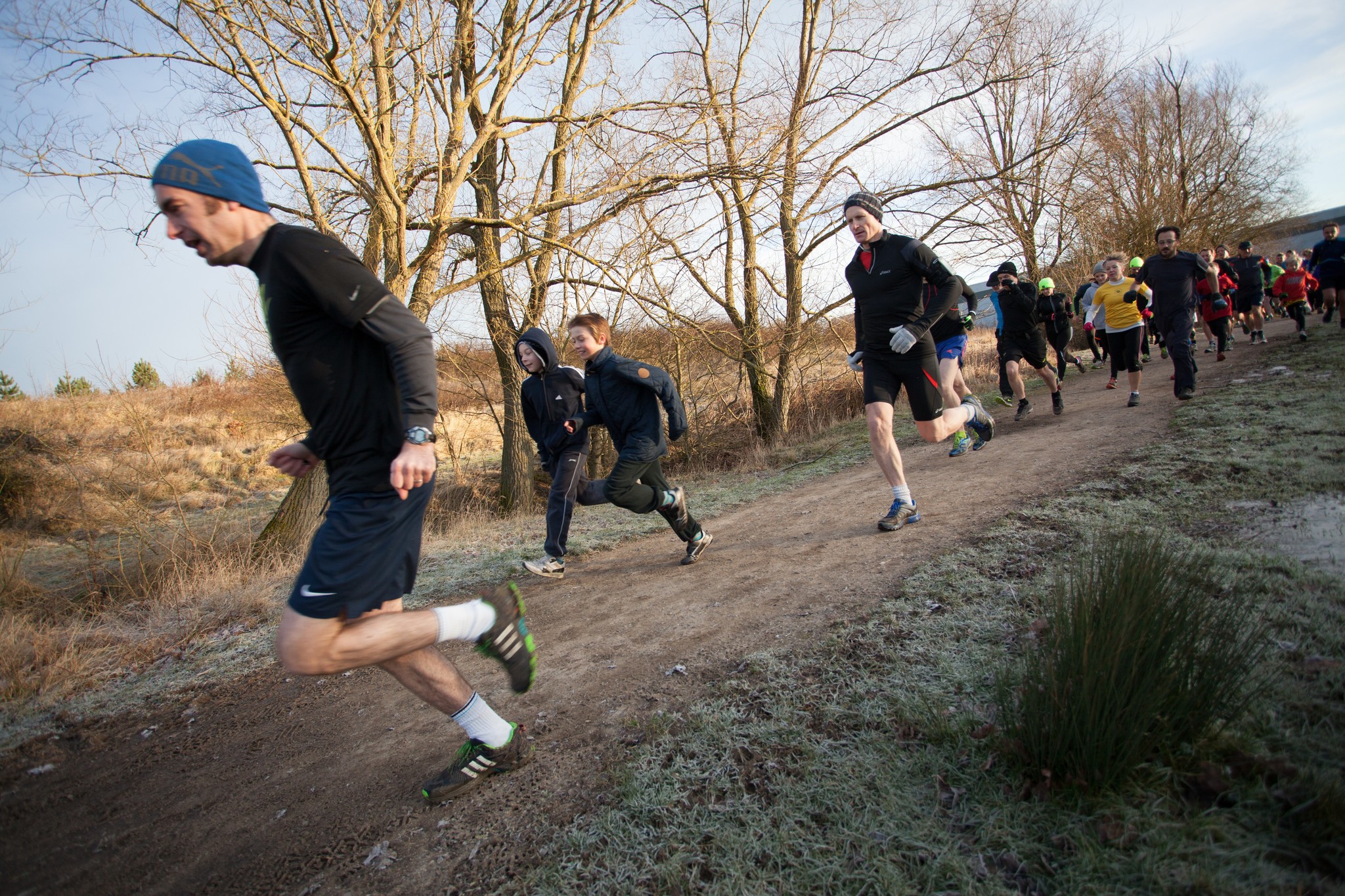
[405,426,436,445]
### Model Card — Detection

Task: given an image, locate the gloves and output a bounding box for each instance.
[1123,290,1137,304]
[994,310,1093,339]
[954,312,975,331]
[1204,288,1231,313]
[846,352,863,373]
[1279,292,1288,299]
[889,325,917,354]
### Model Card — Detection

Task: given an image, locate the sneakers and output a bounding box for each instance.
[416,722,539,803]
[475,582,539,696]
[949,434,972,457]
[960,393,995,442]
[522,553,565,579]
[682,530,714,565]
[995,351,1193,423]
[972,430,987,450]
[661,486,689,530]
[877,498,920,530]
[1138,300,1345,362]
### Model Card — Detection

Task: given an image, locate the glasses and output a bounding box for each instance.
[1158,240,1177,245]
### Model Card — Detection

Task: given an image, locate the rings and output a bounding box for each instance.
[414,480,422,483]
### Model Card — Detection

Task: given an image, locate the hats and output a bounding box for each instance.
[1215,244,1229,252]
[151,139,270,213]
[997,262,1017,279]
[844,191,883,224]
[986,271,1000,287]
[1093,257,1144,278]
[1038,277,1056,291]
[1238,240,1253,250]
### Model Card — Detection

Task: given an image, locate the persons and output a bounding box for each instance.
[987,218,1345,420]
[150,138,539,796]
[562,312,712,565]
[843,191,995,534]
[513,327,615,580]
